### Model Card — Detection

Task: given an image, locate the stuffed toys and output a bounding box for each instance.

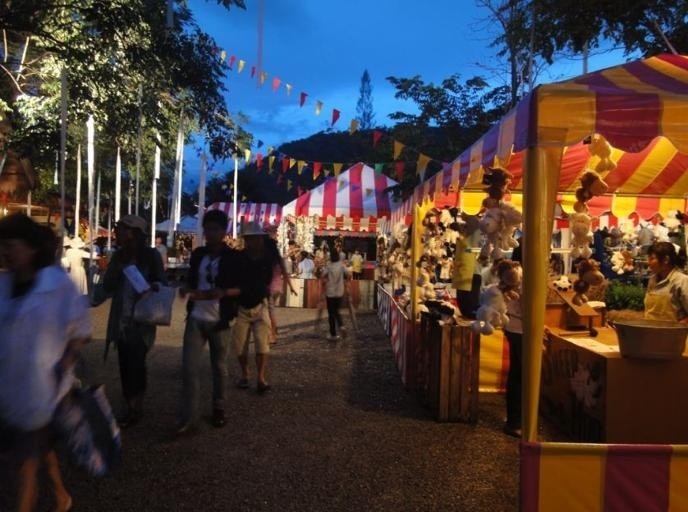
[374,196,523,334]
[572,134,616,307]
[373,136,688,337]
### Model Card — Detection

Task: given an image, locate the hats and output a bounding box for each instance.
[118,214,148,235]
[240,221,269,237]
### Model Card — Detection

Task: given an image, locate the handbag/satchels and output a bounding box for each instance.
[50,383,125,499]
[132,282,176,326]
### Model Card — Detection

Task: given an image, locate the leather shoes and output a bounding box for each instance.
[174,418,192,435]
[257,382,270,391]
[211,406,225,429]
[239,378,250,388]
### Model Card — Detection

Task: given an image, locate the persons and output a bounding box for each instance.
[0,209,95,512]
[318,245,354,342]
[85,214,161,430]
[88,238,107,288]
[281,239,367,279]
[505,236,551,441]
[170,208,247,435]
[643,241,688,324]
[65,237,100,298]
[154,236,169,275]
[234,216,278,394]
[263,239,296,344]
[175,233,184,264]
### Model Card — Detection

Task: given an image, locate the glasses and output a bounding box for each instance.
[204,263,213,283]
